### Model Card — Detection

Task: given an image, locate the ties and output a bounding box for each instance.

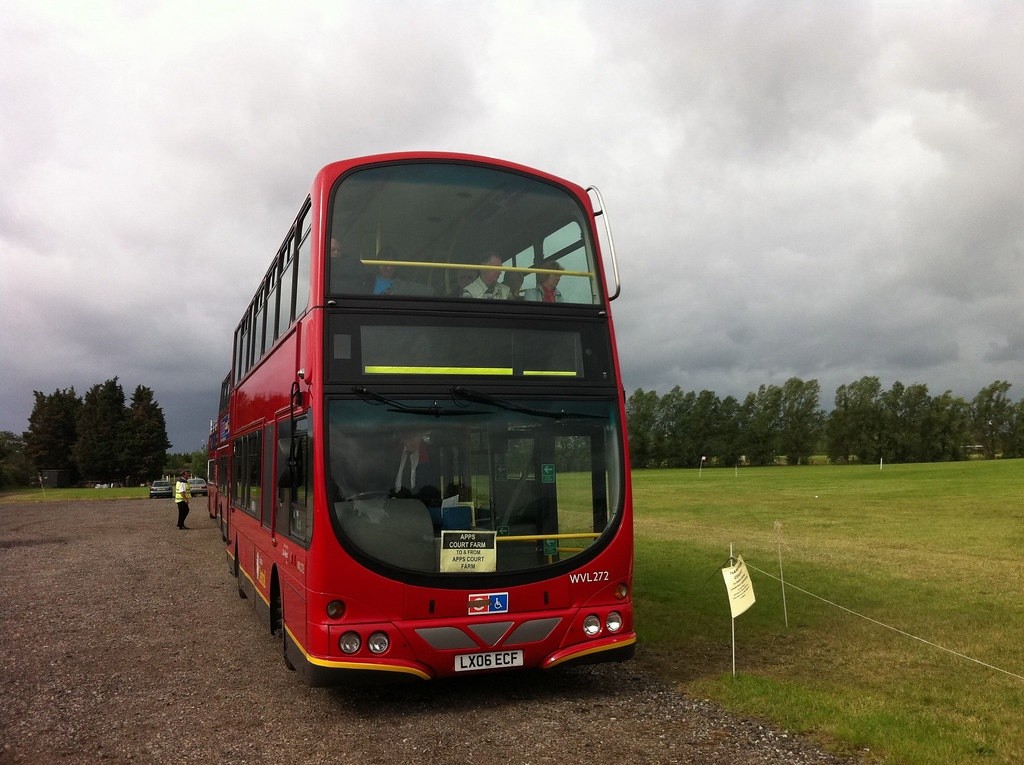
[401,453,411,492]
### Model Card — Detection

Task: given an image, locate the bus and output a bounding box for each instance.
[207,151,637,688]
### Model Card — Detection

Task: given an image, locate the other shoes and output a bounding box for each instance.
[177,525,179,527]
[179,526,190,530]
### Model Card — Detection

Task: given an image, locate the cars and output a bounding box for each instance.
[149,480,173,499]
[187,478,208,497]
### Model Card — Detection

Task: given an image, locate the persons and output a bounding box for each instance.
[502,271,524,298]
[175,470,191,530]
[462,252,512,299]
[523,261,567,303]
[330,238,343,265]
[366,250,403,296]
[390,431,437,505]
[450,270,474,298]
